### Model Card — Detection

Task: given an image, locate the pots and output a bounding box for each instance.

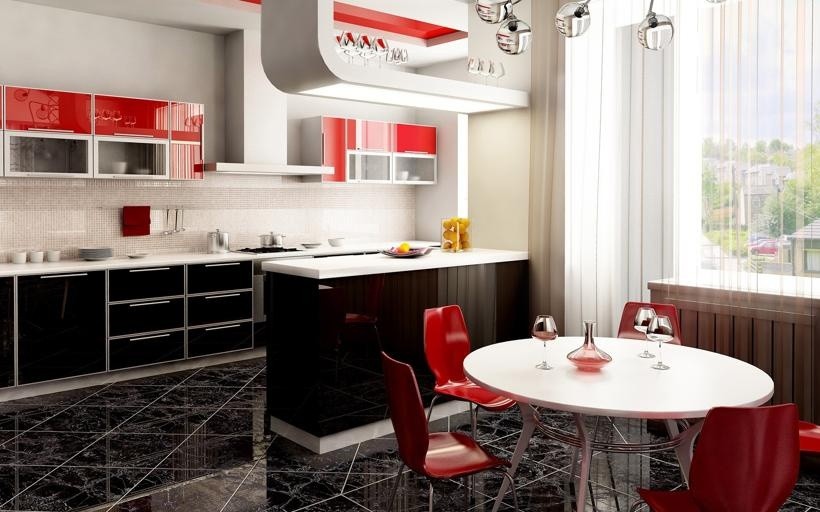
[207,228,230,254]
[257,232,287,248]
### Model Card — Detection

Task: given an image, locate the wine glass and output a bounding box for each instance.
[338,30,390,68]
[468,57,504,87]
[633,307,674,370]
[532,315,558,370]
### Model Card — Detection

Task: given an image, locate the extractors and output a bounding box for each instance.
[205,31,335,176]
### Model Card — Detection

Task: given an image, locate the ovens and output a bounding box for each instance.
[252,256,314,350]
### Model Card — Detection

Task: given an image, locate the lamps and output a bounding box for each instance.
[474,0,675,55]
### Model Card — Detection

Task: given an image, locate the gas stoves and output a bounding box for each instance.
[236,247,303,254]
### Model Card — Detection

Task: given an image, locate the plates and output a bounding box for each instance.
[128,253,151,258]
[410,177,421,180]
[77,248,116,261]
[301,243,322,248]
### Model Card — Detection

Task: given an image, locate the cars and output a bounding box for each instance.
[744,233,779,256]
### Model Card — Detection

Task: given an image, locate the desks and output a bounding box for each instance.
[459,335,775,511]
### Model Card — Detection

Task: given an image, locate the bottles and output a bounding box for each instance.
[567,321,612,373]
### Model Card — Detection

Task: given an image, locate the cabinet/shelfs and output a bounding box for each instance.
[301,116,437,184]
[0,85,204,181]
[0,259,254,402]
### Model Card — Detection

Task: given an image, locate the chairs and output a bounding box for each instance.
[617,301,683,347]
[422,302,518,441]
[797,417,820,456]
[379,350,518,510]
[635,402,798,511]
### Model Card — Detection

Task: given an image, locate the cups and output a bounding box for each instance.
[12,250,60,264]
[112,161,128,173]
[398,170,410,181]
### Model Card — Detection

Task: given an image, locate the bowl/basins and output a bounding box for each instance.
[328,238,347,248]
